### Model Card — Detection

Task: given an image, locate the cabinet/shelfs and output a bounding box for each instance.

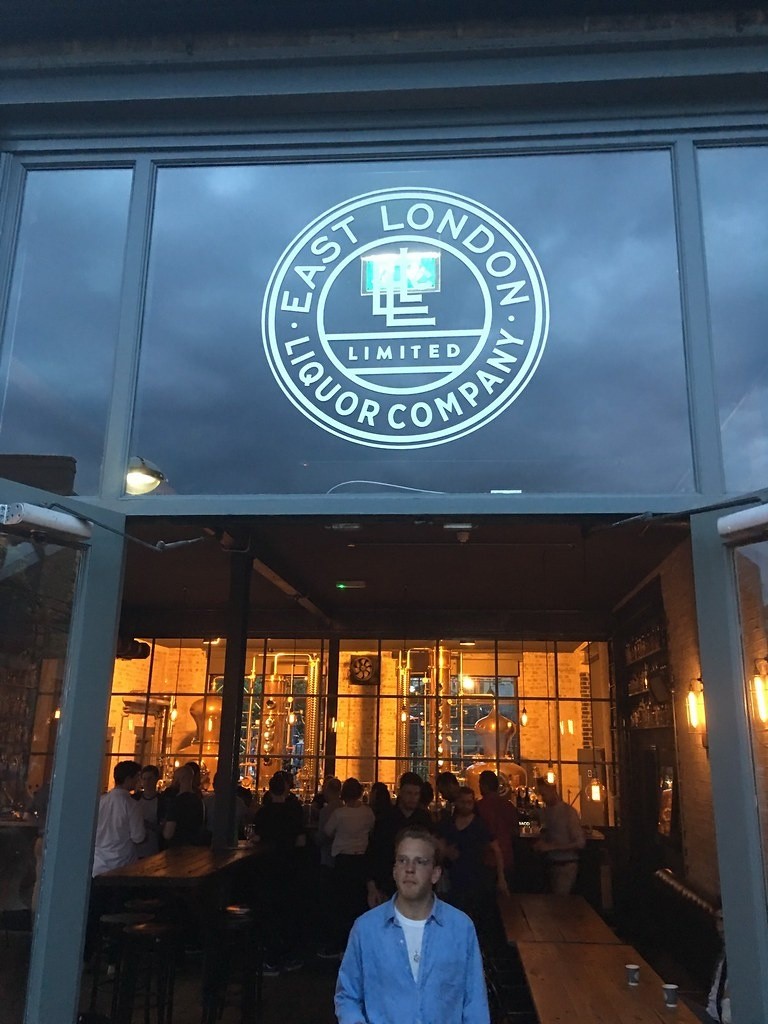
[599,575,676,730]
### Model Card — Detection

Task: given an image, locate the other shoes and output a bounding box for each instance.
[316,947,341,959]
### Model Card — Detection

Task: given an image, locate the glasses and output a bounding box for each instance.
[395,856,438,870]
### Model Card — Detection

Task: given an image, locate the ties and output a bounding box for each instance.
[714,957,727,1024]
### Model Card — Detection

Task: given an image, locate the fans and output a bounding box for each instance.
[349,655,379,686]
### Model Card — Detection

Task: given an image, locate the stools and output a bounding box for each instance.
[90,898,263,1024]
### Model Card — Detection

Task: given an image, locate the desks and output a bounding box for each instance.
[0,818,44,911]
[497,894,703,1024]
[93,844,253,1024]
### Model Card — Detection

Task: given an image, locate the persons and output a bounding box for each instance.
[333,829,491,1023]
[92,762,146,878]
[131,762,585,962]
[32,807,122,982]
[706,896,732,1024]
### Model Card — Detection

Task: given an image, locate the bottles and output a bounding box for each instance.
[623,624,672,728]
[0,780,40,820]
[362,784,368,802]
[516,787,539,816]
[292,784,311,806]
[247,787,266,804]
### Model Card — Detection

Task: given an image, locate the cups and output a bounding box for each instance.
[241,822,257,847]
[625,964,640,986]
[661,984,678,1008]
[519,821,539,834]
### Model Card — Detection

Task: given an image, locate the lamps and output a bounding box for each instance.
[685,677,709,760]
[749,655,768,748]
[123,454,165,496]
